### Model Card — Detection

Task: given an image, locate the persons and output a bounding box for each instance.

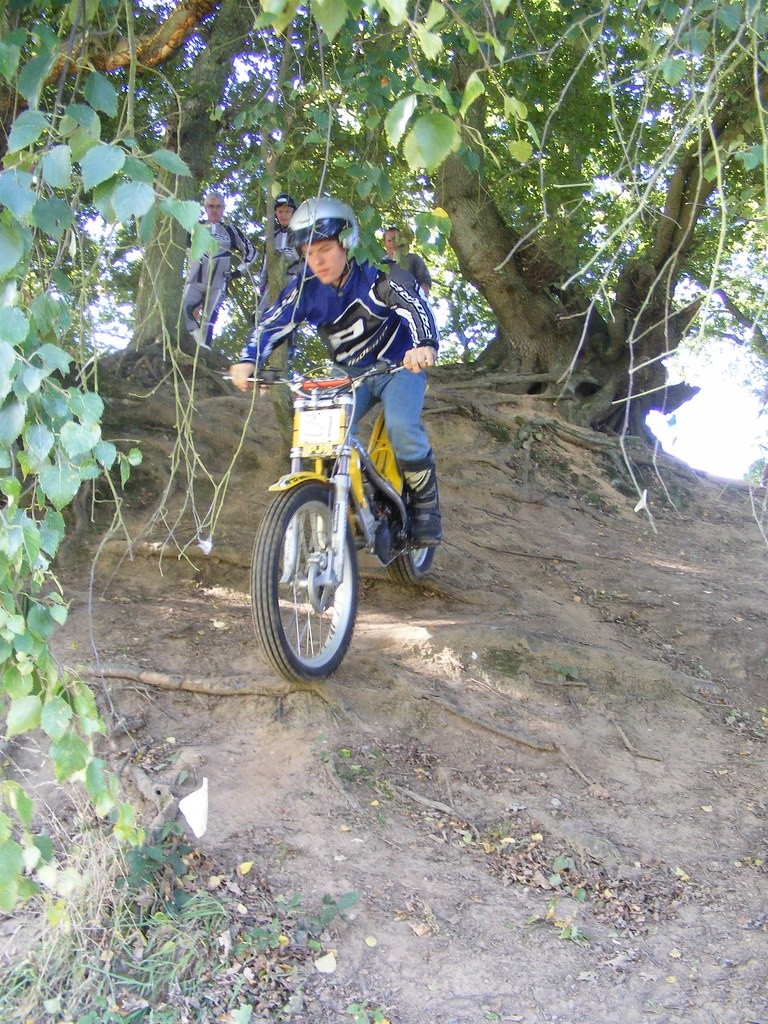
[183,191,257,350]
[223,195,302,380]
[379,227,432,300]
[229,197,441,548]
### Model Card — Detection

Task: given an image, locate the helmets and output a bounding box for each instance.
[274,194,296,212]
[287,196,360,250]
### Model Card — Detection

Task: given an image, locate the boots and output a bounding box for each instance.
[395,450,445,546]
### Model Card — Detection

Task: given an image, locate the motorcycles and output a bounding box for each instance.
[210,359,436,684]
